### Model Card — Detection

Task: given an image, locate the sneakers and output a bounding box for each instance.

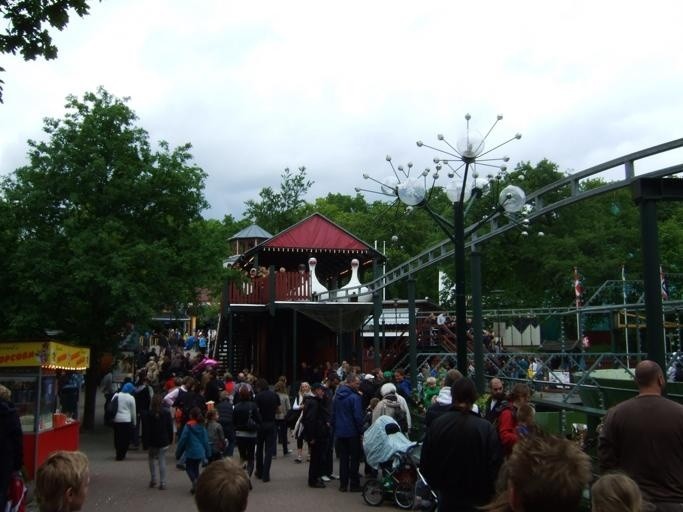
[160,486,165,490]
[255,449,368,492]
[150,479,156,487]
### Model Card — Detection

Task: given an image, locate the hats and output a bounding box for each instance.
[122,383,136,392]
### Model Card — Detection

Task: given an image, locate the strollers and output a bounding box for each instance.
[405,443,438,512]
[360,412,422,510]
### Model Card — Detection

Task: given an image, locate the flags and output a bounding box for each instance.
[581,318,589,347]
[660,269,668,301]
[574,275,584,303]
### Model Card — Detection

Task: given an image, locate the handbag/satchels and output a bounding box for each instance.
[107,396,118,419]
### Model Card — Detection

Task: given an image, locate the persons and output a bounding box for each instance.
[1,330,559,512]
[222,261,306,294]
[598,360,683,511]
[590,474,642,511]
[107,382,137,460]
[302,382,329,489]
[174,407,211,494]
[478,431,592,511]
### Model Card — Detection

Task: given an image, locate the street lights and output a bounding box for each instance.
[353,109,545,376]
